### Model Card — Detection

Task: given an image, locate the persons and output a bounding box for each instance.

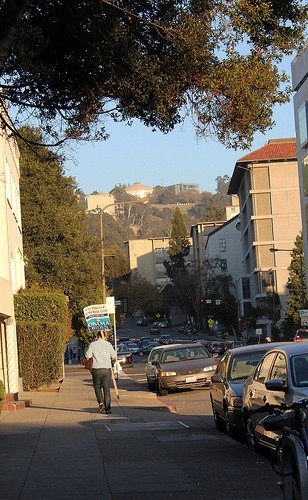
[84,331,116,414]
[70,346,81,362]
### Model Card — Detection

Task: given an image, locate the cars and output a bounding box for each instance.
[241,342,308,455]
[294,329,308,343]
[151,343,219,396]
[146,344,190,392]
[210,341,296,440]
[110,322,233,368]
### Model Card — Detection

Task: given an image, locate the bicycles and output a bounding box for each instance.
[240,398,308,500]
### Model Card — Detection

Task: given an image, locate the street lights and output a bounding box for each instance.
[100,199,150,342]
[268,248,304,309]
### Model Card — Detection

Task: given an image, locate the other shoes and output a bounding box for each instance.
[102,409,110,414]
[99,403,105,413]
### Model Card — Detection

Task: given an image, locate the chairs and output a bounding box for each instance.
[232,360,254,376]
